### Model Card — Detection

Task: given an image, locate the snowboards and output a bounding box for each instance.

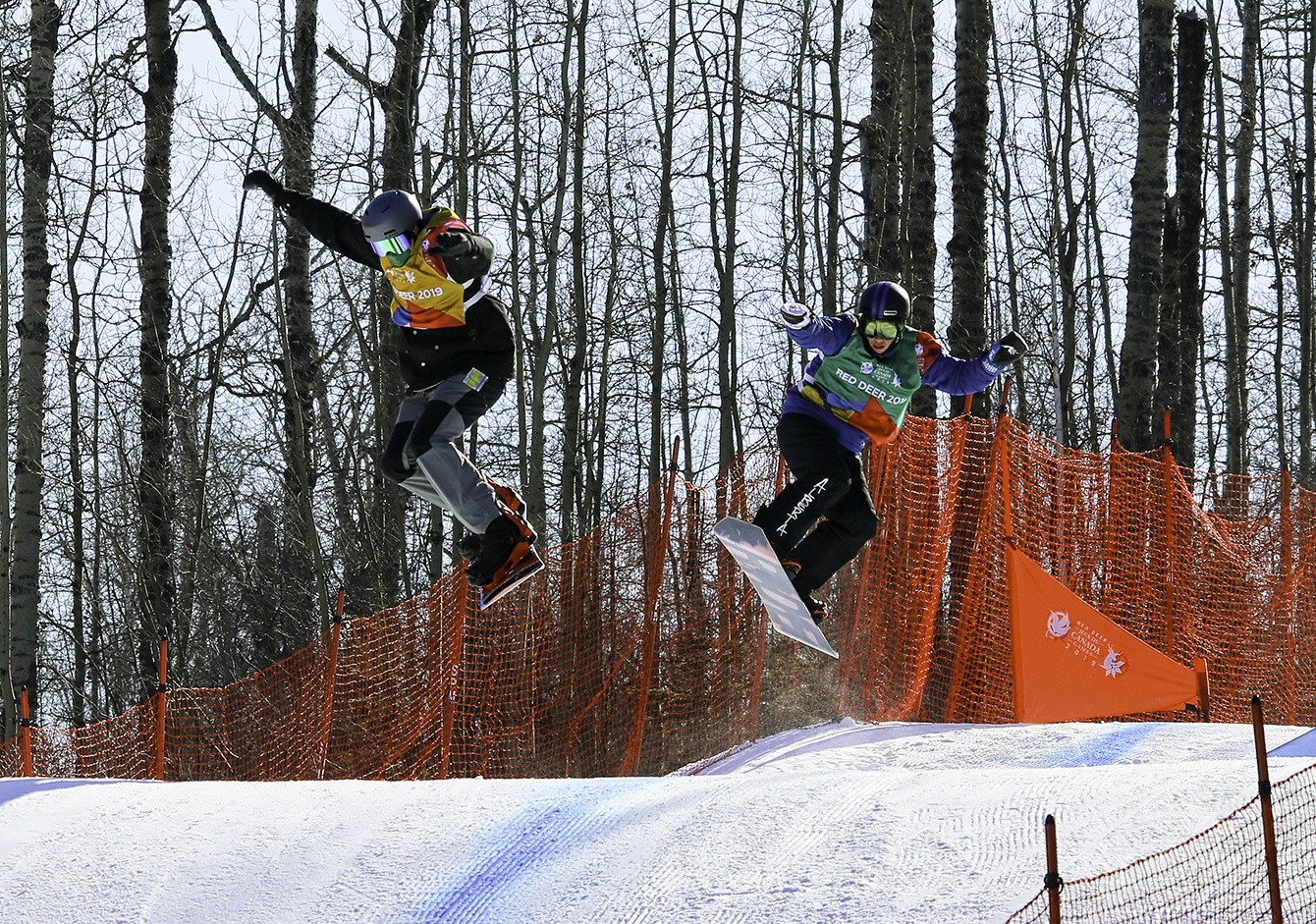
[478,521,546,611]
[713,517,839,659]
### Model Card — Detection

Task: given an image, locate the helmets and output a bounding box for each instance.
[857,281,910,322]
[362,190,422,242]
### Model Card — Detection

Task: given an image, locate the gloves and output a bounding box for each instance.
[242,170,287,208]
[428,231,469,257]
[981,331,1029,376]
[779,303,814,330]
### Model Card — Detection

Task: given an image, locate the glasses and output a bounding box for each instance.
[862,320,898,340]
[370,229,413,258]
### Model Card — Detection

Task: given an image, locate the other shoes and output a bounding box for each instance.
[780,557,801,578]
[799,592,828,625]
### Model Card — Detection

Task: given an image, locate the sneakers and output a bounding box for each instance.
[455,487,526,562]
[463,499,538,588]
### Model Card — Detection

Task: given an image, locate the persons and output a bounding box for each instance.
[754,280,1028,623]
[243,171,522,587]
